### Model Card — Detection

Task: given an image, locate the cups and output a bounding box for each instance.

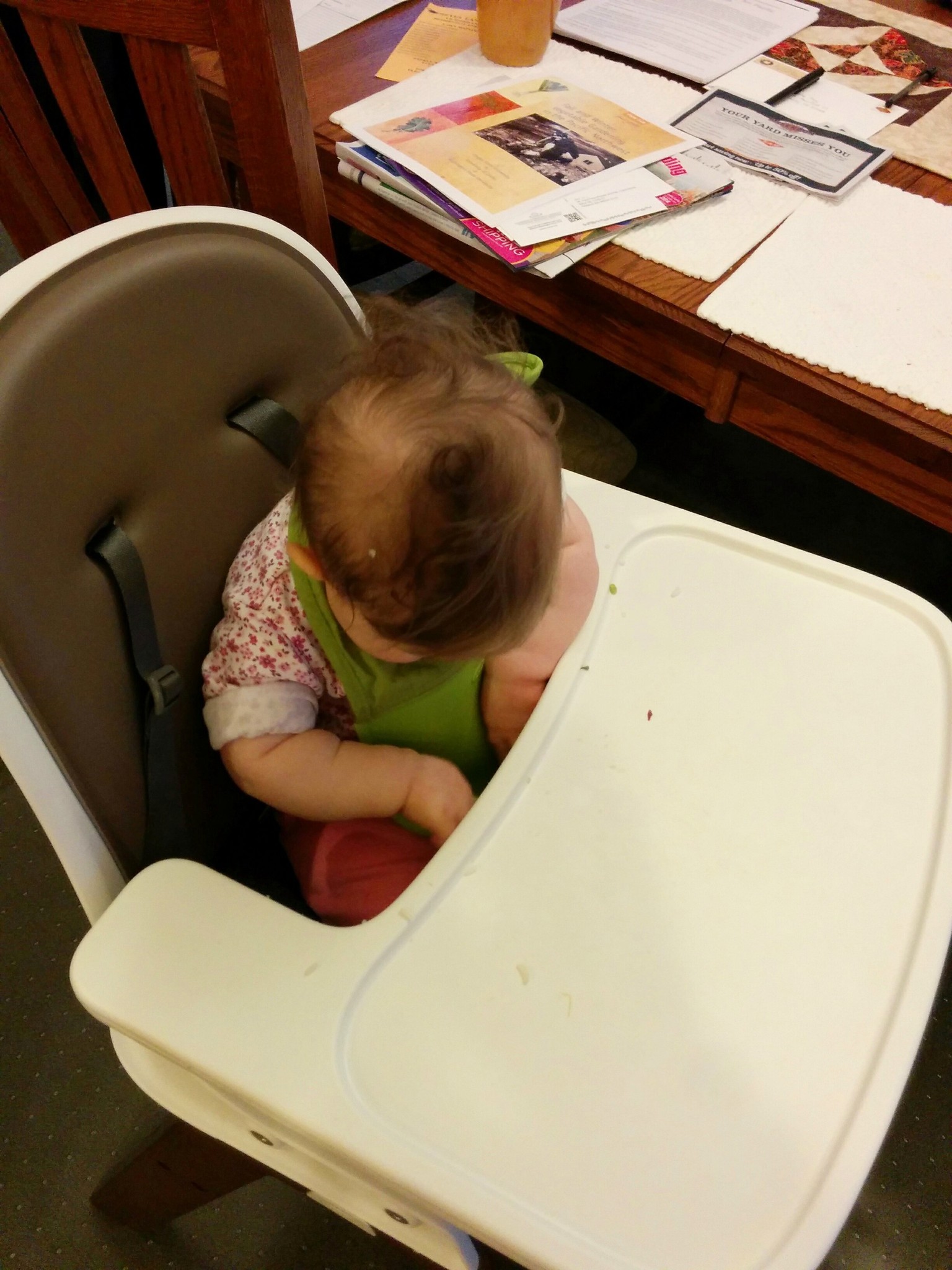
[475,0,562,68]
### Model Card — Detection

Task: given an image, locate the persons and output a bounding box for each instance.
[200,303,598,921]
[523,131,580,161]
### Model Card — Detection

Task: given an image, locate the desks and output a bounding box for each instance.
[185,0,952,536]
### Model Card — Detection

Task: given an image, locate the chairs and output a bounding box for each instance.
[0,0,514,337]
[0,205,952,1270]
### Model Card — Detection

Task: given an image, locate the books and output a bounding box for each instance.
[328,72,735,279]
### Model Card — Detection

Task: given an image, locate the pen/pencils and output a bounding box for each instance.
[765,66,825,107]
[885,67,937,109]
